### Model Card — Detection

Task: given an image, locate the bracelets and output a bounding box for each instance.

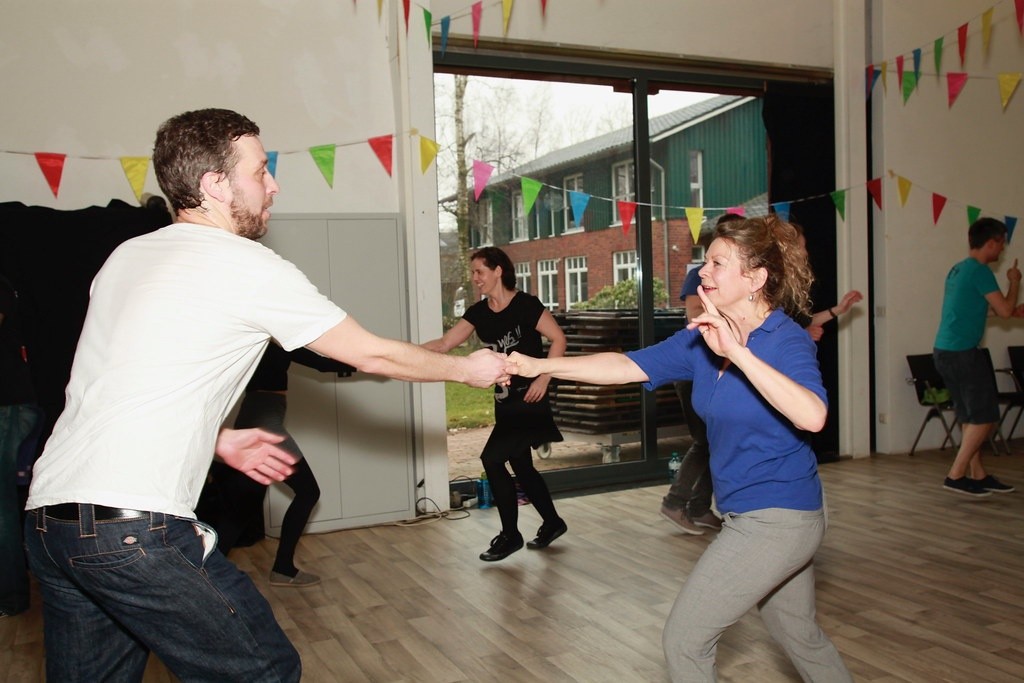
[829,308,837,318]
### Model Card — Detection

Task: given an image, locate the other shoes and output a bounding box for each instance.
[479,530,524,561]
[659,503,705,536]
[971,474,1015,493]
[268,570,321,587]
[942,475,993,498]
[526,517,567,550]
[690,509,722,531]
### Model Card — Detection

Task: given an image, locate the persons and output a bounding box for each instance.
[19,108,517,683]
[420,246,567,561]
[214,341,362,586]
[505,215,853,683]
[658,214,862,534]
[933,217,1024,497]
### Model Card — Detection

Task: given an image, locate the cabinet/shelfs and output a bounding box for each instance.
[258,213,416,535]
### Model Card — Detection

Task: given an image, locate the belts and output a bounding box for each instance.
[32,502,149,521]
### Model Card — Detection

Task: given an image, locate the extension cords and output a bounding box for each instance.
[463,498,478,507]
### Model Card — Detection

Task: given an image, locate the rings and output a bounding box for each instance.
[701,329,709,335]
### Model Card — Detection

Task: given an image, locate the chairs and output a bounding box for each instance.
[905,345,1024,458]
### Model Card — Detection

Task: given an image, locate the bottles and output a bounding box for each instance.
[478,472,492,510]
[668,453,683,483]
